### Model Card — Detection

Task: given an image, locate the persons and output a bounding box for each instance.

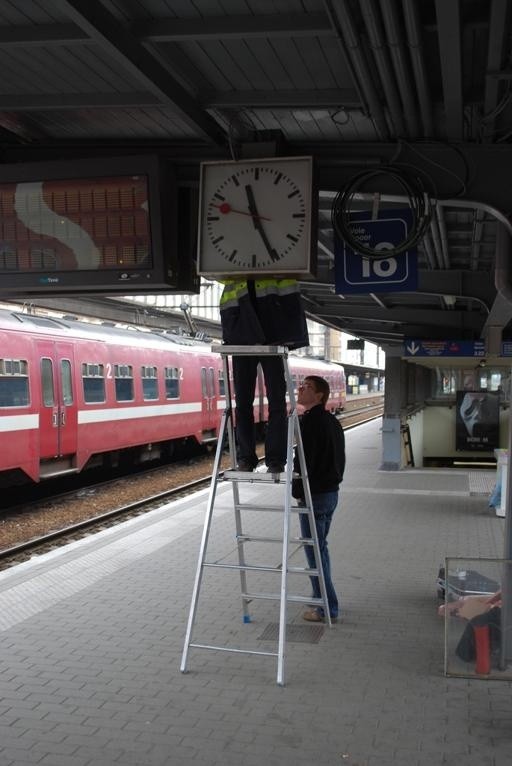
[219,279,309,474]
[292,374,346,620]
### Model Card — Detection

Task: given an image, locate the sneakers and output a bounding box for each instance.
[238,465,253,472]
[267,465,284,473]
[302,605,337,623]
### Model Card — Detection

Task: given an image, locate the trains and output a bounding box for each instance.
[0,309,346,483]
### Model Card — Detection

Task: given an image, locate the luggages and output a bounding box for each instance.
[437,568,499,601]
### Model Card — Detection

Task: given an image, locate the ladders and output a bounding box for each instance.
[180,345,332,686]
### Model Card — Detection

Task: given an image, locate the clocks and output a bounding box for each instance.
[197,156,319,280]
